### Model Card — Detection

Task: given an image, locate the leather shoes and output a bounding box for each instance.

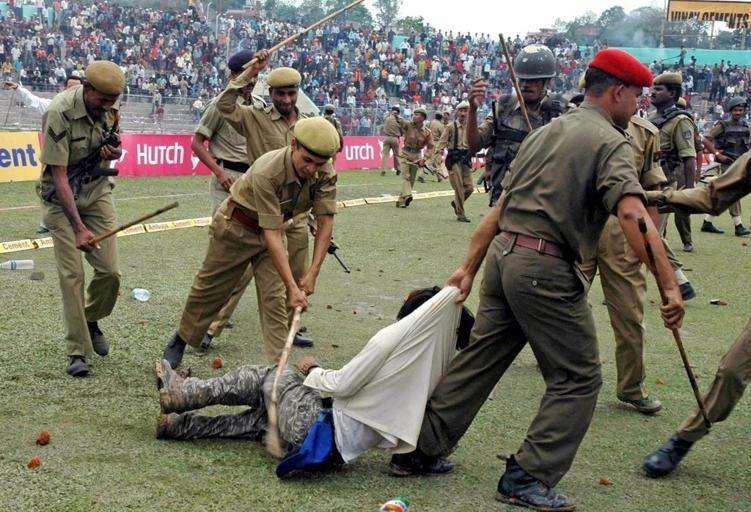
[86,321,109,356]
[292,333,315,348]
[156,411,180,441]
[65,354,90,377]
[494,453,578,511]
[199,333,215,350]
[153,357,185,415]
[701,219,725,234]
[641,431,696,479]
[735,223,751,237]
[615,391,663,415]
[682,242,694,252]
[388,444,455,477]
[223,319,235,329]
[163,332,187,370]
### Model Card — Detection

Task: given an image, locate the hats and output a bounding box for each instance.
[227,50,256,72]
[587,47,653,88]
[265,66,302,88]
[652,72,682,86]
[85,60,126,96]
[414,108,427,120]
[455,100,469,109]
[293,116,341,160]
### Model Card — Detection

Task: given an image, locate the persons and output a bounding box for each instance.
[155,285,476,479]
[434,102,473,223]
[389,108,435,208]
[192,49,344,357]
[191,49,269,223]
[35,61,125,376]
[646,72,696,237]
[381,107,406,175]
[646,44,696,107]
[253,13,464,107]
[551,40,608,95]
[0,0,223,126]
[389,49,685,512]
[3,76,84,234]
[700,97,750,236]
[417,111,445,182]
[643,151,750,479]
[697,57,750,120]
[465,43,579,205]
[161,118,339,371]
[323,104,384,142]
[465,32,548,121]
[579,64,668,412]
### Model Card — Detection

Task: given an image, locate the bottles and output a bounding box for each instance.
[131,287,148,302]
[378,496,411,512]
[1,259,34,270]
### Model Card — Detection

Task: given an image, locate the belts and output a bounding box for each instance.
[228,205,261,230]
[76,168,119,184]
[214,158,249,175]
[496,229,563,257]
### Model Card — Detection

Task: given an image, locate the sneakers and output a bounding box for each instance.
[457,216,470,223]
[395,201,405,207]
[405,196,413,206]
[678,282,697,302]
[451,201,458,215]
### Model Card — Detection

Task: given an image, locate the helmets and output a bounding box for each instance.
[513,43,557,80]
[728,97,747,112]
